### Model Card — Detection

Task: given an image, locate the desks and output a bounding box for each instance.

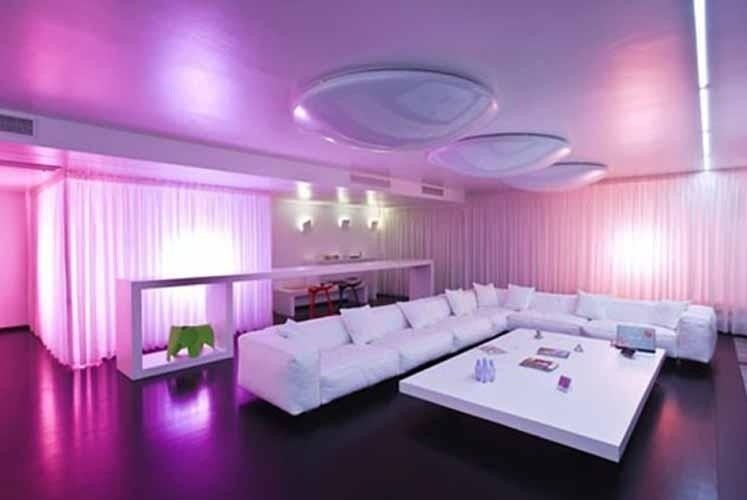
[115,257,435,381]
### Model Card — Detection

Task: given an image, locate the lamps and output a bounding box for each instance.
[291,66,500,151]
[302,218,378,233]
[426,134,571,179]
[499,164,611,194]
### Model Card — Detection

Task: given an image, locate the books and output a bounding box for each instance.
[519,357,559,371]
[537,346,570,359]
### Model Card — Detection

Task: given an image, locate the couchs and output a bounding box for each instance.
[273,275,368,318]
[237,288,717,416]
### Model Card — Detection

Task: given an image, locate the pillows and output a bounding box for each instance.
[446,282,689,328]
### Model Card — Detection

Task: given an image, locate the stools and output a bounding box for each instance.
[334,276,362,311]
[308,281,334,319]
